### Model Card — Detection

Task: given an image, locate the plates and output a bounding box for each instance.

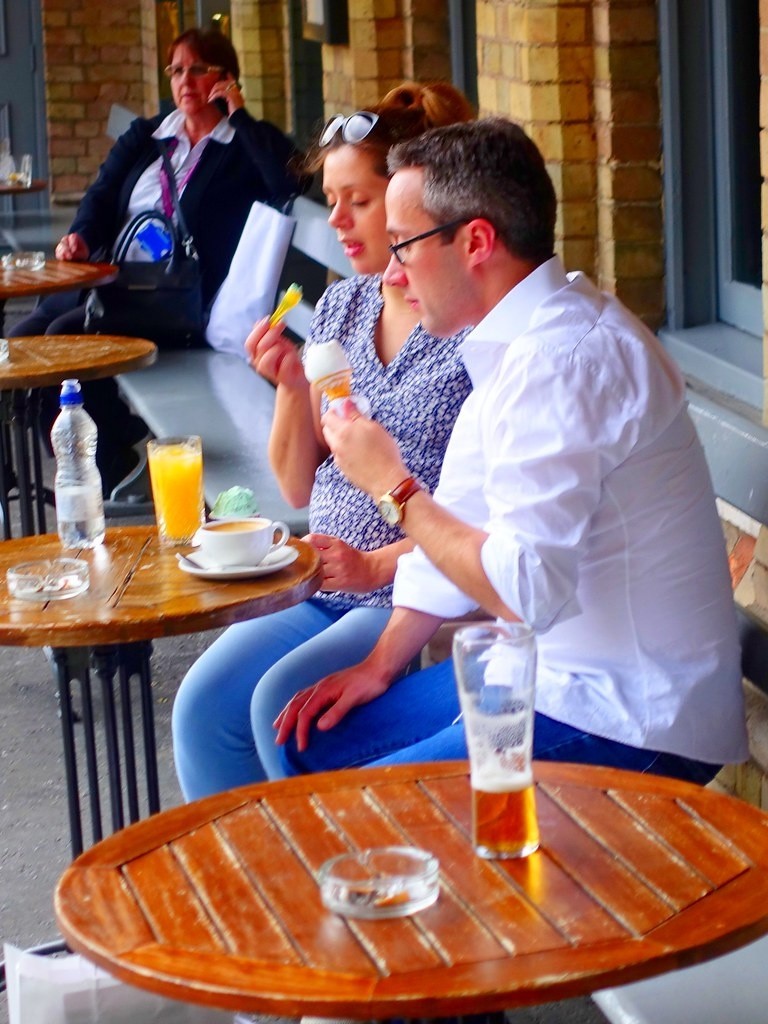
[176,545,299,579]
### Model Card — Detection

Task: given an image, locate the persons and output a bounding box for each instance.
[167,79,472,796]
[272,116,749,791]
[8,27,314,500]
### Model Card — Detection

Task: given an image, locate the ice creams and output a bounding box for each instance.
[208,486,261,521]
[304,339,351,403]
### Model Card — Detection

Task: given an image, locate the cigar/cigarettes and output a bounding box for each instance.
[60,241,70,252]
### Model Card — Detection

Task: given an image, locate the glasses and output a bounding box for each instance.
[165,61,229,78]
[390,211,498,263]
[317,110,403,147]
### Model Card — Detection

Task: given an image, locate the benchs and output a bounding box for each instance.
[83,104,768,1023]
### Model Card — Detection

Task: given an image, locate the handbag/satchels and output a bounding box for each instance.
[85,209,202,341]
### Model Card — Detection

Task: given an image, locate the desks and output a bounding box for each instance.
[0,525,323,991]
[0,262,119,338]
[53,763,767,1023]
[0,335,158,536]
[0,179,49,194]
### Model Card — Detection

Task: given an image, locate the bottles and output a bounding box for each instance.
[50,380,106,551]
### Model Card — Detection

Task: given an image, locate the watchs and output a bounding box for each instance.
[378,475,423,529]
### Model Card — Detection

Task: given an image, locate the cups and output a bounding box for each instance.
[146,434,207,548]
[200,517,290,568]
[452,619,541,861]
[7,154,33,187]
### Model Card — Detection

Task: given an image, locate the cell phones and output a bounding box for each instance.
[214,78,242,116]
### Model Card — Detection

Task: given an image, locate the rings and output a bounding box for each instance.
[225,80,237,91]
